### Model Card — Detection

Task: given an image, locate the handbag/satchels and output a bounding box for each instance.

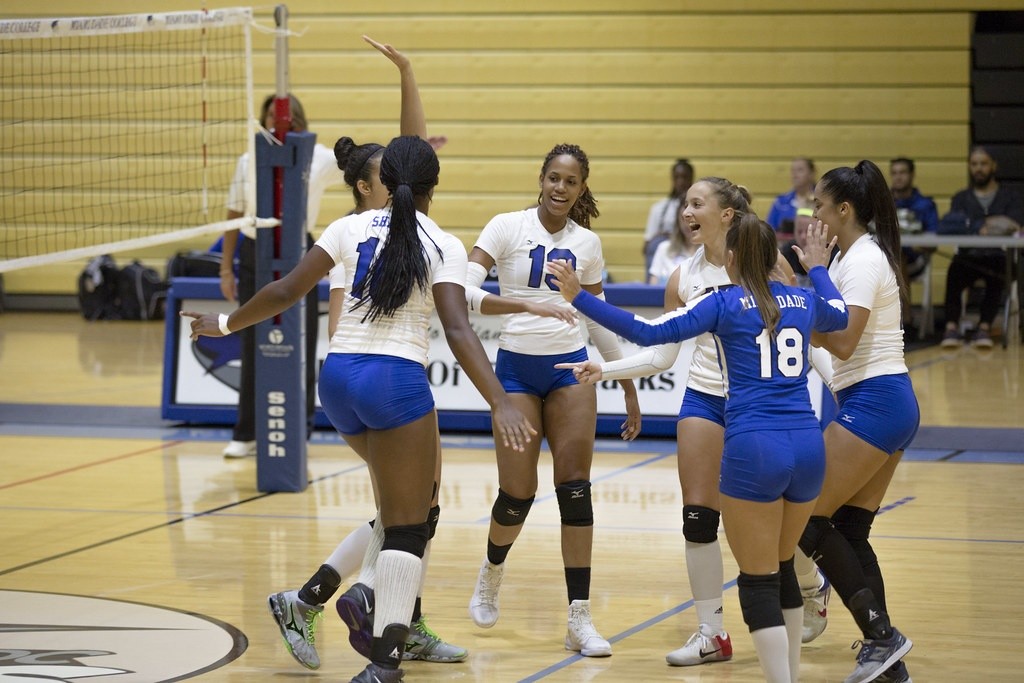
[78,253,165,322]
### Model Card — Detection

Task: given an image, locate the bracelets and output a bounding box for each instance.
[217,314,232,336]
[218,270,233,276]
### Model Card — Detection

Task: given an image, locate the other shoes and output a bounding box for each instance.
[941,328,959,348]
[974,329,993,348]
[223,437,256,459]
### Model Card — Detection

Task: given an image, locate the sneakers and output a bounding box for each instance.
[565,599,612,657]
[266,588,325,671]
[470,556,506,629]
[345,623,406,683]
[666,631,733,666]
[799,567,831,643]
[841,627,913,683]
[400,613,468,662]
[336,582,375,658]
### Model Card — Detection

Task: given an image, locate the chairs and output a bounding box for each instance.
[903,253,1018,349]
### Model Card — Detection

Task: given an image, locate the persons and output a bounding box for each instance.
[266,37,466,672]
[225,92,447,458]
[458,144,1024,683]
[180,138,538,683]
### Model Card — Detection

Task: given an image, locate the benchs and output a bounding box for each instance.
[0,1,970,316]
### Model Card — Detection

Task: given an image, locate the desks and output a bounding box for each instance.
[158,274,838,434]
[776,233,1024,352]
